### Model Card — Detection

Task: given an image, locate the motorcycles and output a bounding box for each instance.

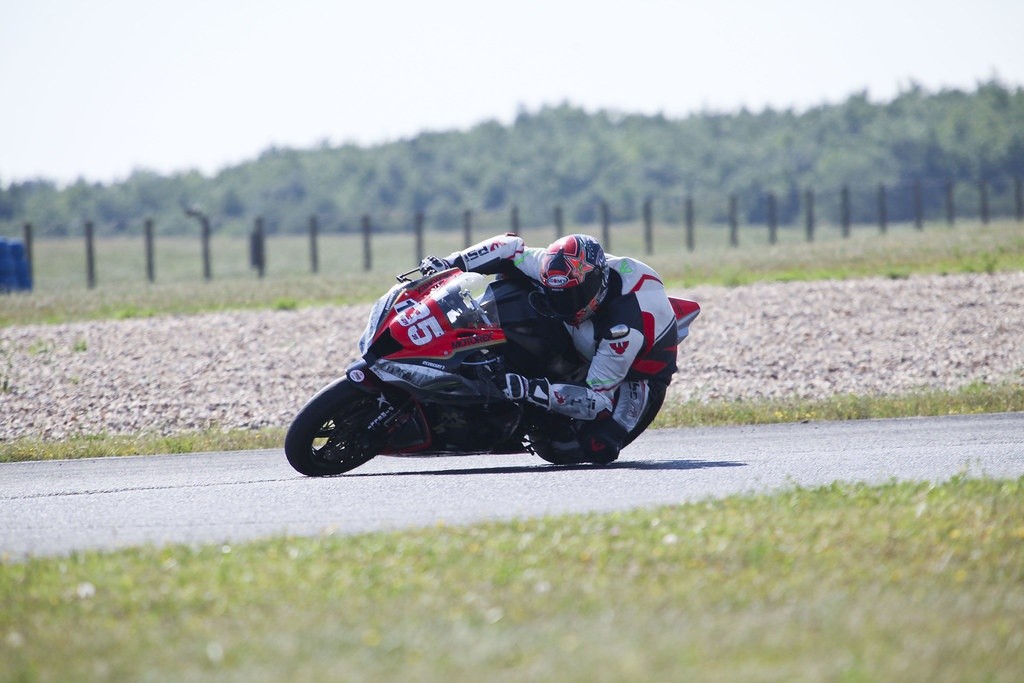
[283,263,701,475]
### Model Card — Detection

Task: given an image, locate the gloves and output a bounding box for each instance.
[418,251,465,277]
[498,373,550,412]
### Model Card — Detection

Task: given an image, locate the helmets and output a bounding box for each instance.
[540,233,607,327]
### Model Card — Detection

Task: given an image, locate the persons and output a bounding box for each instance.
[419,233,676,465]
